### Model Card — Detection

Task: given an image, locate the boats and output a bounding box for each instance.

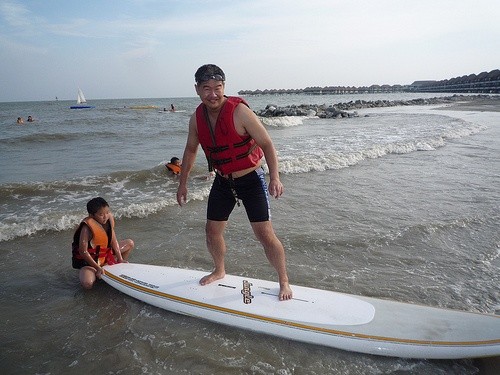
[130,106,161,109]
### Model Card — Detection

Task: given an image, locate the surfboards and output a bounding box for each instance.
[99,262,500,359]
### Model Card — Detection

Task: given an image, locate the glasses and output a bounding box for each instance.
[197,74,225,81]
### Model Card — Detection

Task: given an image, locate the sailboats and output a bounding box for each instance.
[69,87,93,109]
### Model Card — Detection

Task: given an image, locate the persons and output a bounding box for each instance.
[77,197,134,289]
[170,104,175,111]
[176,64,292,301]
[17,117,23,124]
[168,157,182,179]
[27,116,33,122]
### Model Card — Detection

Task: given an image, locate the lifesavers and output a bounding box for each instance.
[166,163,182,175]
[196,96,263,175]
[72,214,115,269]
